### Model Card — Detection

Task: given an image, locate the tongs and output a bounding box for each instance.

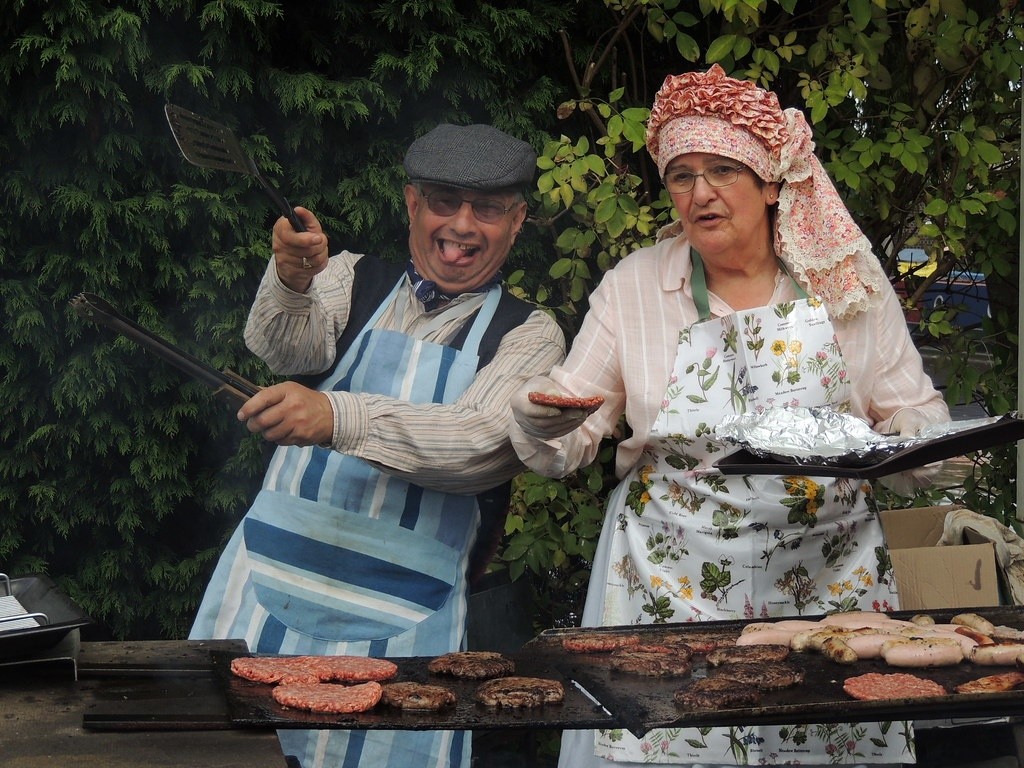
[68,291,264,410]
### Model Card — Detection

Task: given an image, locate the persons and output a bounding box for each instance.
[509,70,951,768]
[186,123,566,768]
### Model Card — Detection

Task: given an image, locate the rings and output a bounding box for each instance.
[303,257,312,269]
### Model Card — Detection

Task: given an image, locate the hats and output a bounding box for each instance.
[404,124,539,187]
[642,63,884,319]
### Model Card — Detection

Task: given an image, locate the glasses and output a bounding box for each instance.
[418,189,522,225]
[664,161,748,196]
